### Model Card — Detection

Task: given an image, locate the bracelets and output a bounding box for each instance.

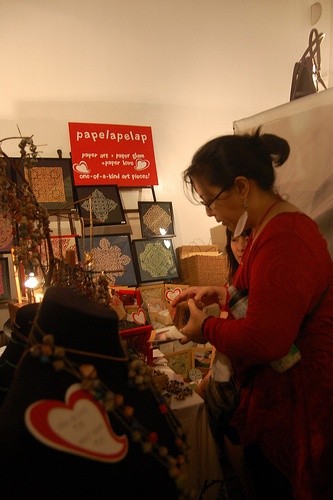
[200,316,214,337]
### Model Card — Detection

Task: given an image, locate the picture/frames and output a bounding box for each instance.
[75,185,126,227]
[137,201,176,238]
[132,237,179,282]
[15,156,75,211]
[75,232,139,287]
[139,284,164,308]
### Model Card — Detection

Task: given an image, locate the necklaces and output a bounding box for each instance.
[3,322,197,500]
[241,193,282,262]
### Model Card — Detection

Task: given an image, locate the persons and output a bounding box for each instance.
[171,126,333,500]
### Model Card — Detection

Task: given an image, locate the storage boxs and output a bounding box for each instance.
[175,243,219,265]
[182,255,228,287]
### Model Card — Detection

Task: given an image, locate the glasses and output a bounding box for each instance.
[200,186,229,210]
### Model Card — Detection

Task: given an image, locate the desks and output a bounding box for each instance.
[151,348,224,500]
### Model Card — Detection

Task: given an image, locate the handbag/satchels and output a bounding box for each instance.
[290,29,328,101]
[203,377,240,430]
